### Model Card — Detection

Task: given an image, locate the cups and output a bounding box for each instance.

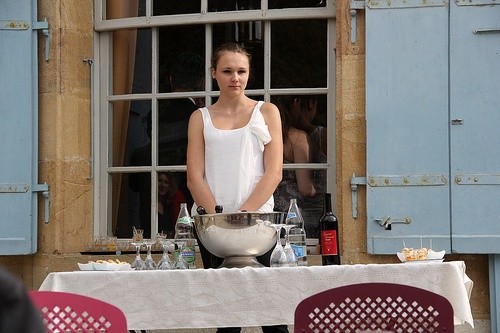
[132,229,144,243]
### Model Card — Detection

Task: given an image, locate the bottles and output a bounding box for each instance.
[316,193,341,265]
[196,205,208,216]
[215,205,224,214]
[175,203,196,269]
[285,199,307,266]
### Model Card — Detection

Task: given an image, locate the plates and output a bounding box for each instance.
[78,261,131,271]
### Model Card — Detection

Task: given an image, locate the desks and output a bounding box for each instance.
[37,261,474,333]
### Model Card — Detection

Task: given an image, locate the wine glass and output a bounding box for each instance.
[280,224,298,268]
[269,223,287,267]
[131,240,189,269]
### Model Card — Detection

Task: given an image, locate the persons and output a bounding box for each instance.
[156,173,177,238]
[291,94,328,239]
[146,53,317,236]
[187,41,289,333]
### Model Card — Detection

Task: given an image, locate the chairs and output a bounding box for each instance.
[294,282,455,333]
[24,291,128,333]
